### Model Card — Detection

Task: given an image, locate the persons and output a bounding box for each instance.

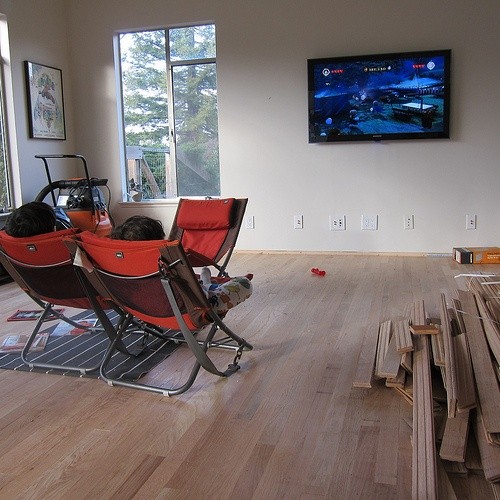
[109,215,255,301]
[6,201,58,240]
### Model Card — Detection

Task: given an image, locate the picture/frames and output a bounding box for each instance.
[24,60,67,140]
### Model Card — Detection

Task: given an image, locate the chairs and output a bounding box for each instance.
[62,231,254,397]
[0,227,156,375]
[168,198,248,278]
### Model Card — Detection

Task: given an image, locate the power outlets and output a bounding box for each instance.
[294,215,303,229]
[466,215,476,229]
[330,216,346,231]
[404,215,414,230]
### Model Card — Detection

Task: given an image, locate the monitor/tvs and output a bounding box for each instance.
[308,49,451,144]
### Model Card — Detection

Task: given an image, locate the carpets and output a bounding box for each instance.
[0,307,199,383]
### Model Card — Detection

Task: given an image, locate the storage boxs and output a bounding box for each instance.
[452,247,500,264]
